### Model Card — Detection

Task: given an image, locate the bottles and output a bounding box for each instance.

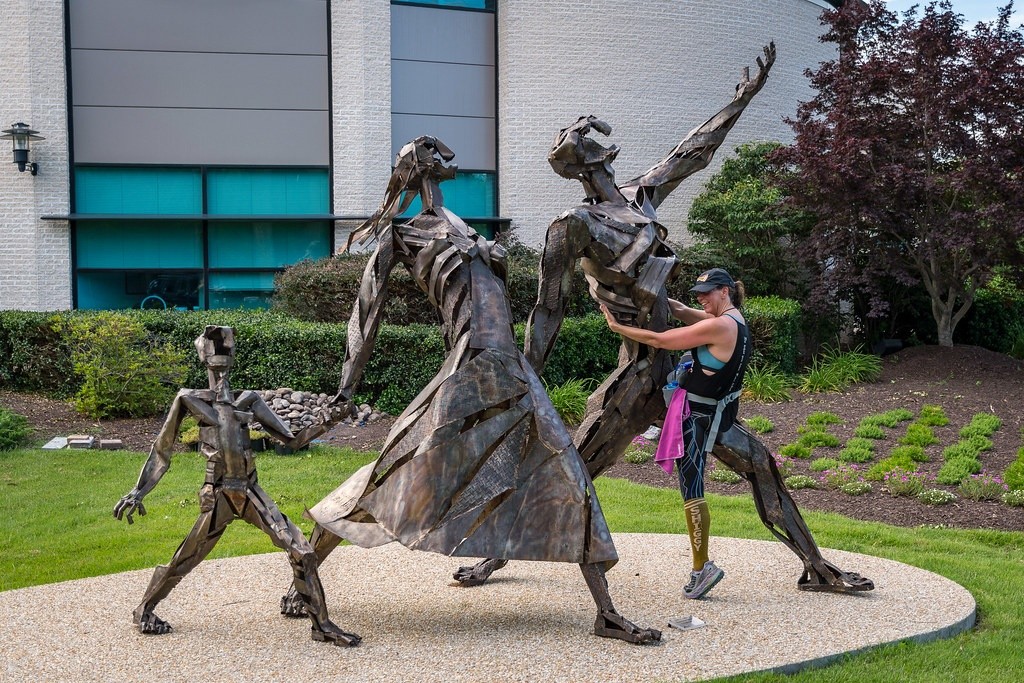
[681,361,692,370]
[667,381,678,389]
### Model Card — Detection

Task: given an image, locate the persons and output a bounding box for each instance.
[599,267,750,599]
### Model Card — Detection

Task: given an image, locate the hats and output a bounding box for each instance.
[687,268,735,292]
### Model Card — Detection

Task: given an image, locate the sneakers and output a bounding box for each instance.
[683,559,724,598]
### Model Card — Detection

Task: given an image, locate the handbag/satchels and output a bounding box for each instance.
[662,351,697,408]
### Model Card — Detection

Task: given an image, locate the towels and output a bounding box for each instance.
[653,388,690,475]
